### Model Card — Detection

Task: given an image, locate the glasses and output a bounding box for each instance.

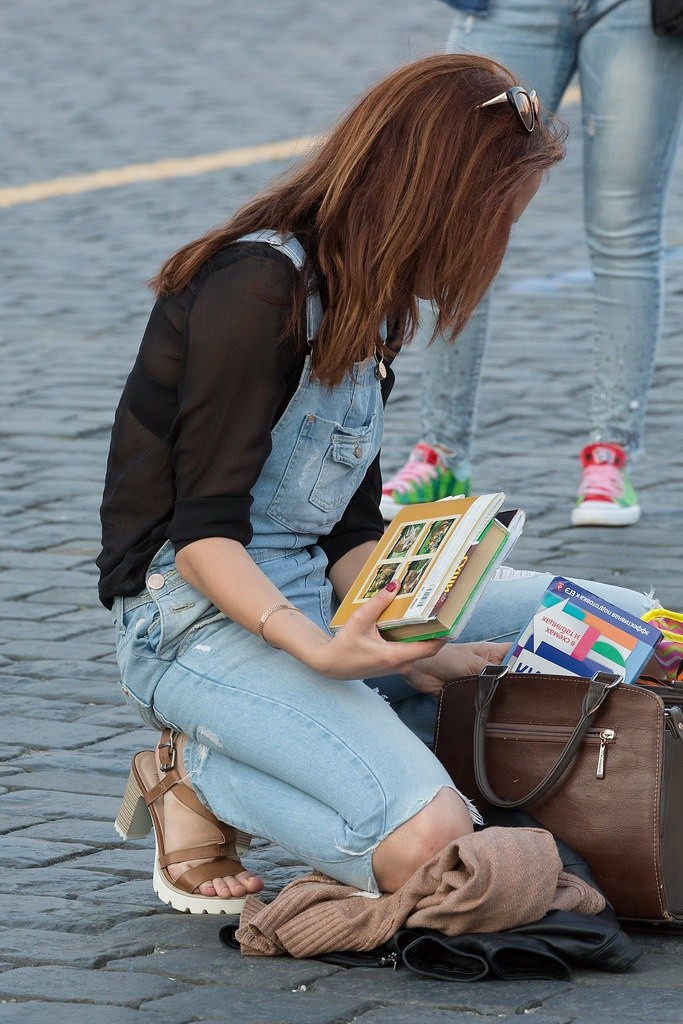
[475,86,543,133]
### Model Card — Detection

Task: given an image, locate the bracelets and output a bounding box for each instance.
[257,604,304,650]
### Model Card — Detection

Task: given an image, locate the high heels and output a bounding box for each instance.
[114,728,262,915]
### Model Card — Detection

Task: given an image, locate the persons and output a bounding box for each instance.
[379,0,683,525]
[94,52,664,915]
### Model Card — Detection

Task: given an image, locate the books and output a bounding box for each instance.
[500,575,665,685]
[328,492,526,642]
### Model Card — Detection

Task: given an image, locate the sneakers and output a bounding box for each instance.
[571,443,640,525]
[378,440,472,521]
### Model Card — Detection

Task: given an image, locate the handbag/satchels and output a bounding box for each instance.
[433,666,683,932]
